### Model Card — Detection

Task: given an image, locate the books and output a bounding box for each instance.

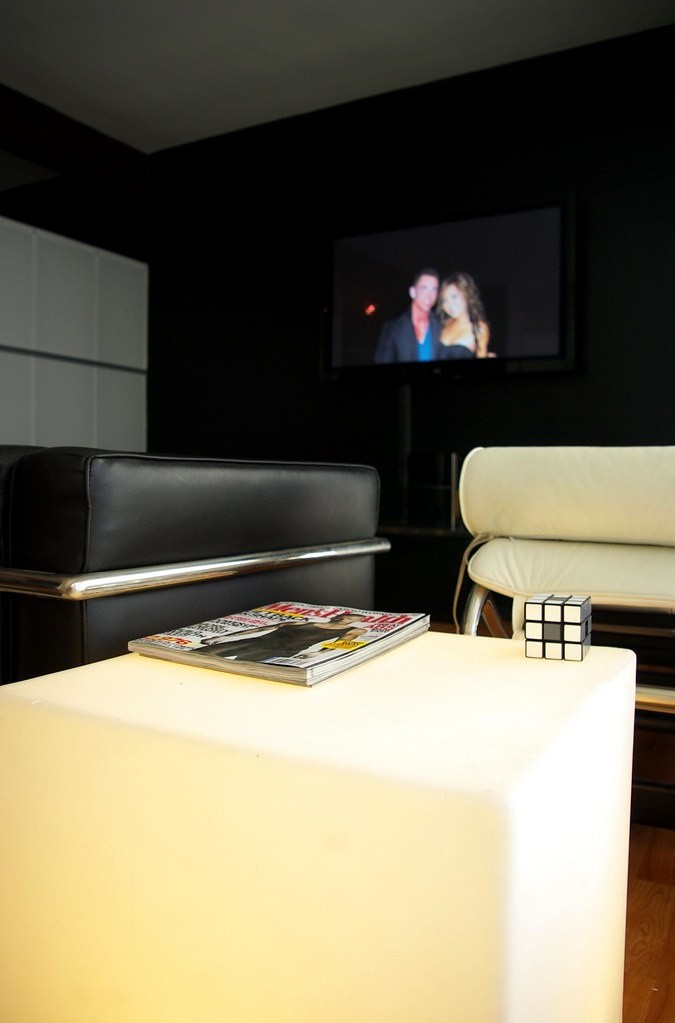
[127,600,430,688]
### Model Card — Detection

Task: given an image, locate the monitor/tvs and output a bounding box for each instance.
[328,203,582,385]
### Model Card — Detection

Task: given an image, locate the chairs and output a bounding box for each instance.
[0,442,392,686]
[452,445,675,717]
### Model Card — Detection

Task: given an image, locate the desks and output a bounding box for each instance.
[0,631,637,1023]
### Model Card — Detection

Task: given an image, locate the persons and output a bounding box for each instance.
[435,270,489,360]
[375,270,442,362]
[191,614,367,662]
